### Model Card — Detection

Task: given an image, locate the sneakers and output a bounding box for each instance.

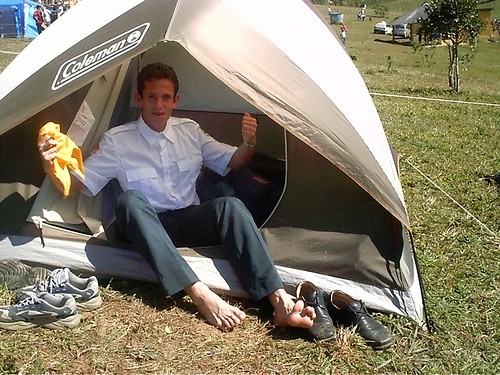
[0,292,79,330]
[16,269,102,311]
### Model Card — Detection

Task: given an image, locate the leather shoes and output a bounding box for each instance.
[296,281,336,342]
[329,290,396,350]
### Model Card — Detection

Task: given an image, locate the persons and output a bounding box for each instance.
[34,5,67,34]
[340,23,347,45]
[38,63,316,328]
[327,6,364,20]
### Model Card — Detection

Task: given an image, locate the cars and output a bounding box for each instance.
[393,24,410,38]
[374,23,388,34]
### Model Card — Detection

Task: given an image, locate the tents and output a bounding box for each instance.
[0,0,40,38]
[0,0,434,333]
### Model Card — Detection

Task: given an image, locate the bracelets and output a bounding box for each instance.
[245,142,256,148]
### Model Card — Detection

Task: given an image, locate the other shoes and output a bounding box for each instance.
[0,259,37,290]
